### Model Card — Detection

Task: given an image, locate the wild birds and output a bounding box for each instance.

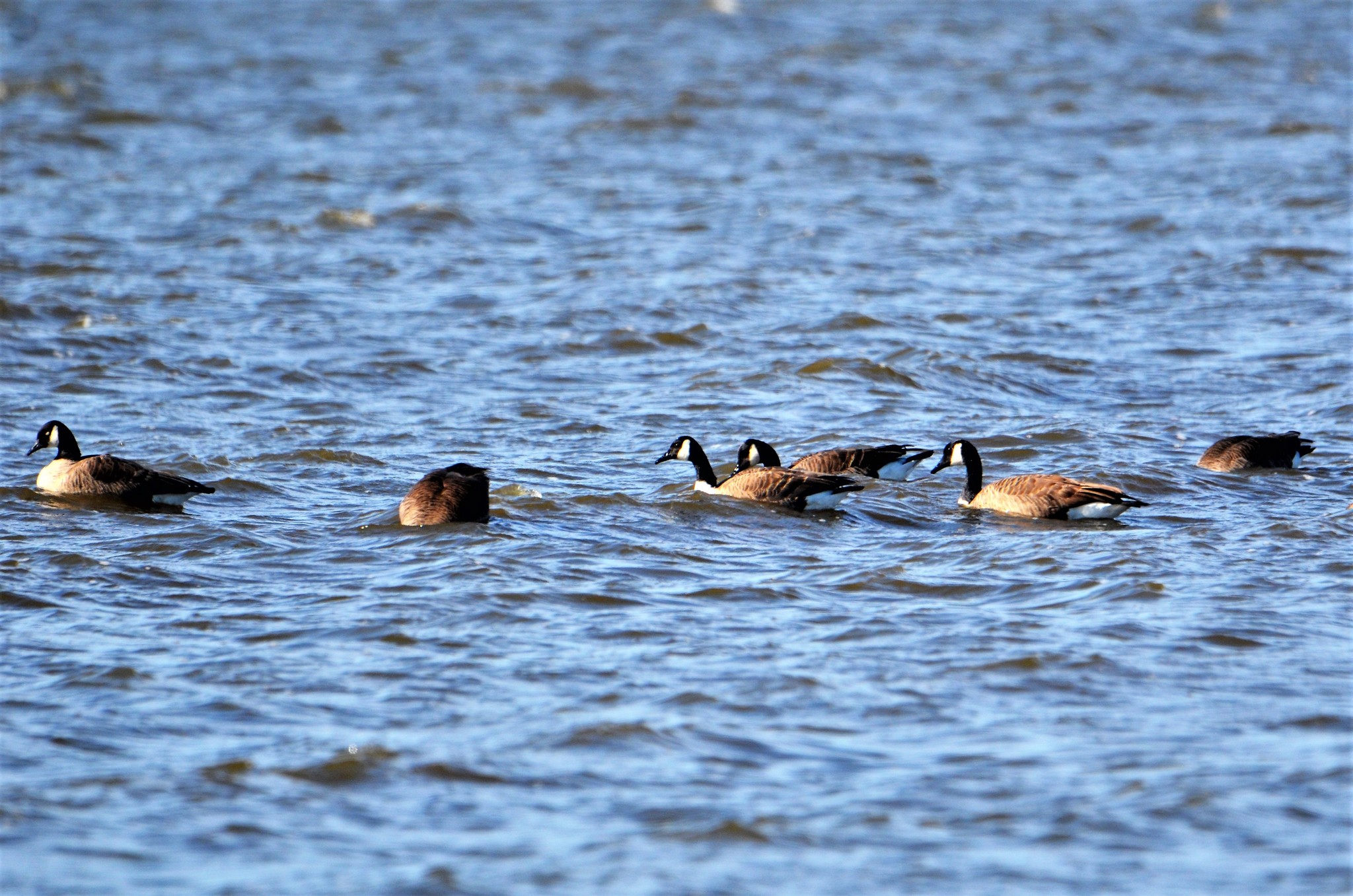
[1195,431,1317,473]
[654,435,935,510]
[931,440,1151,522]
[26,420,216,505]
[399,463,490,526]
